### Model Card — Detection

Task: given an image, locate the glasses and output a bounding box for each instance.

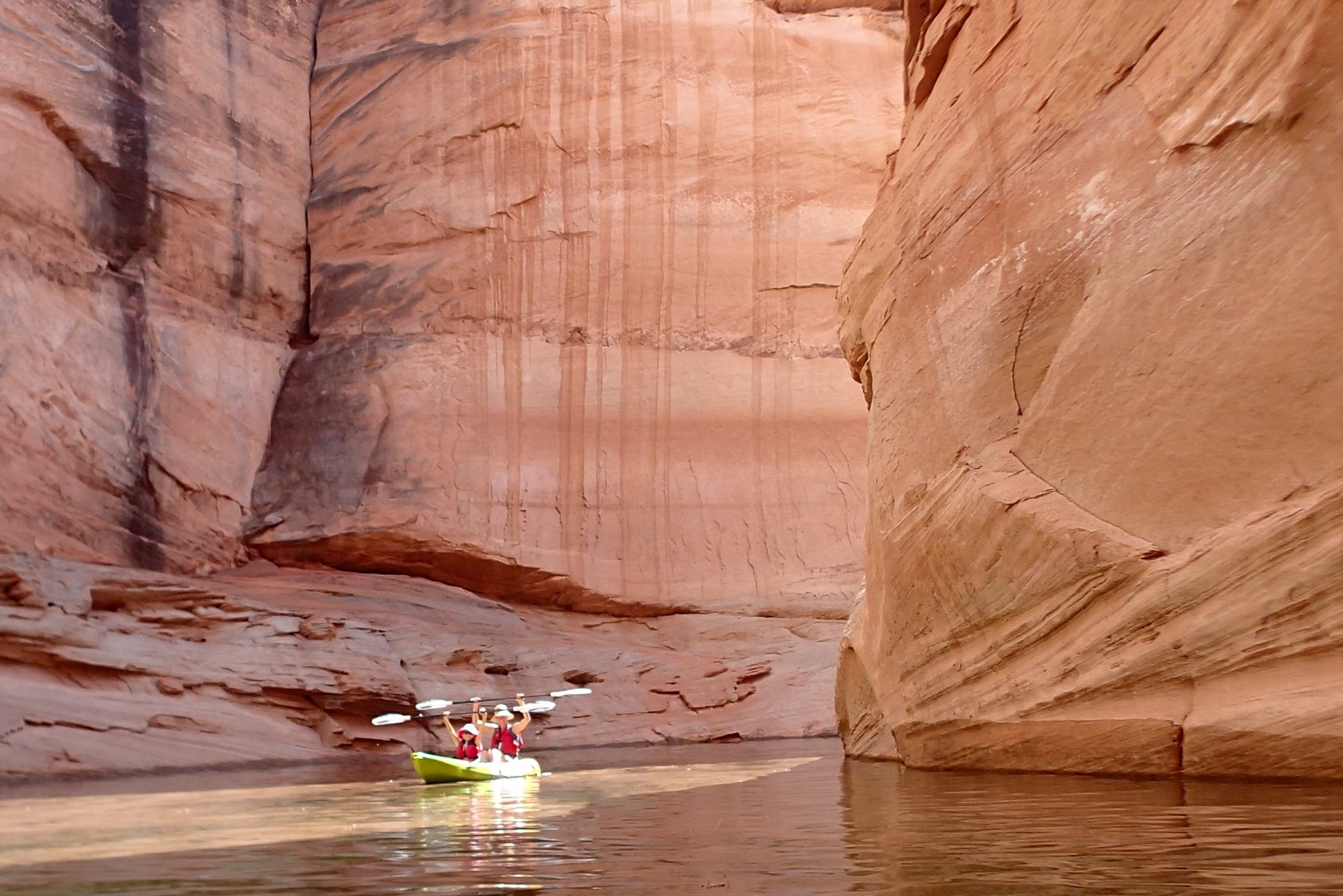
[464,731,472,734]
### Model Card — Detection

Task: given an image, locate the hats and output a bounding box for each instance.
[490,704,514,723]
[458,723,479,739]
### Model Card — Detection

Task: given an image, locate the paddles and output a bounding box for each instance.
[373,700,557,726]
[415,688,592,711]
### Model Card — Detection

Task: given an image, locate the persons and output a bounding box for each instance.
[472,693,531,762]
[443,708,487,761]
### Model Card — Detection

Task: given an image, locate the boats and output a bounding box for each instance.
[411,751,542,785]
